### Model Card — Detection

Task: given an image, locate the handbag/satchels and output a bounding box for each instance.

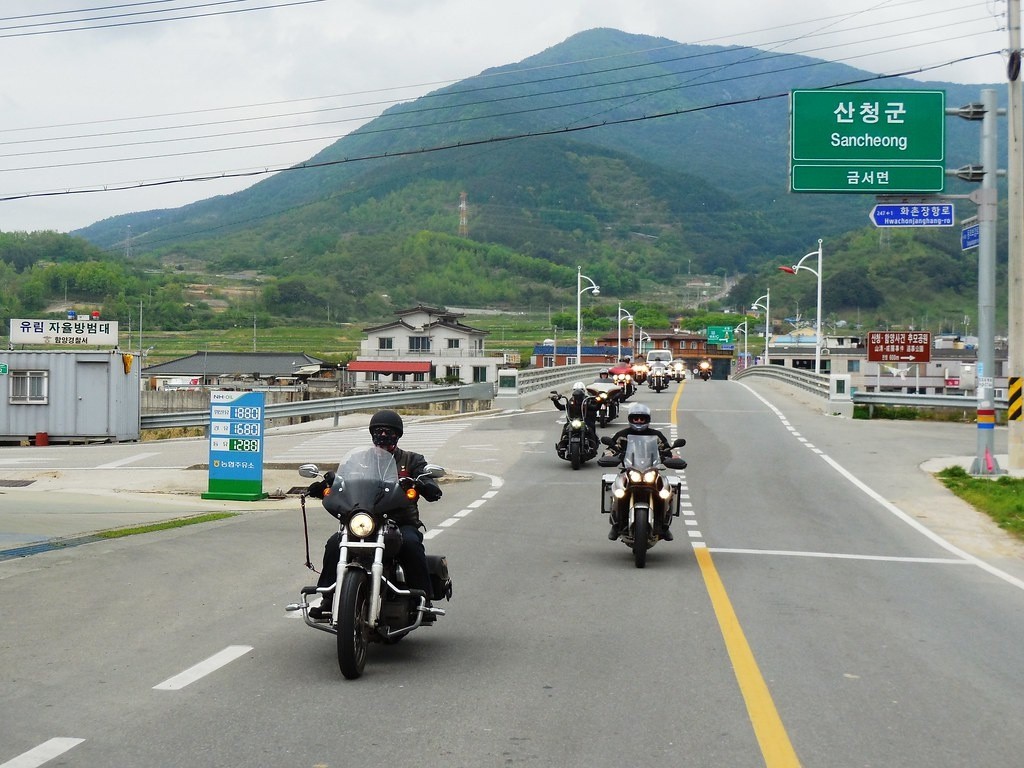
[422,481,442,502]
[425,555,453,601]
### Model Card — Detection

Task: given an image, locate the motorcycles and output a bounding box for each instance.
[548,390,608,471]
[647,363,670,393]
[600,433,688,571]
[697,361,712,381]
[631,361,649,386]
[585,382,623,428]
[671,361,687,384]
[607,361,638,404]
[284,462,445,684]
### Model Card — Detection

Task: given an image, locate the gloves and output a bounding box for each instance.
[307,479,328,497]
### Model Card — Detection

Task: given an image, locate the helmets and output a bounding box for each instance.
[369,410,404,438]
[572,382,586,404]
[655,357,660,362]
[624,355,630,362]
[627,403,651,431]
[599,368,609,378]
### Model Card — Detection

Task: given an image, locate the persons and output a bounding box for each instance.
[601,404,679,542]
[307,408,443,620]
[551,356,713,451]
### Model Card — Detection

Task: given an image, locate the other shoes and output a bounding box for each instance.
[422,603,437,622]
[607,523,619,540]
[308,597,332,619]
[662,525,673,540]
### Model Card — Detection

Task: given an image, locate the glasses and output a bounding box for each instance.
[374,427,396,436]
[634,417,646,421]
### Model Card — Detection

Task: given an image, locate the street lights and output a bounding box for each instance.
[735,317,748,369]
[576,265,600,365]
[751,288,770,365]
[617,303,634,362]
[778,238,822,374]
[639,327,651,355]
